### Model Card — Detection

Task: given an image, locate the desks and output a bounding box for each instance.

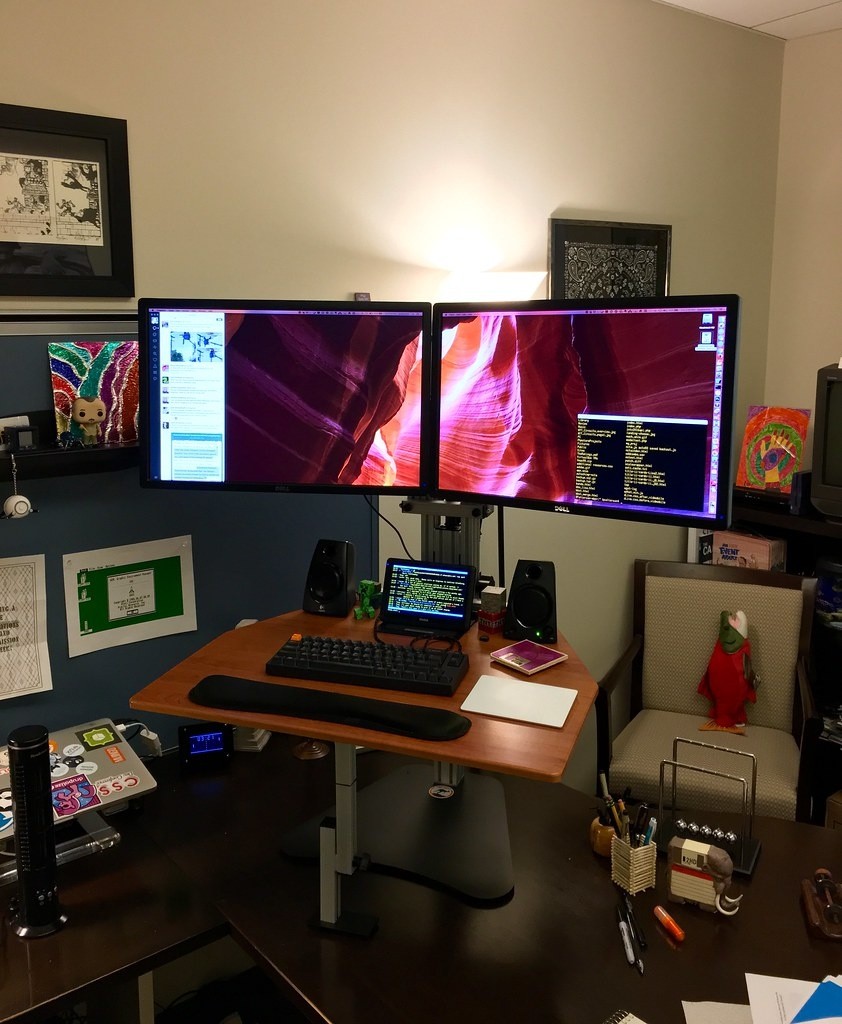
[0,733,842,1024]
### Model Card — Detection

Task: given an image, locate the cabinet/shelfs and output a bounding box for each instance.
[687,485,842,829]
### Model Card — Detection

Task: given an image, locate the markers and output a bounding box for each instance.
[616,908,636,964]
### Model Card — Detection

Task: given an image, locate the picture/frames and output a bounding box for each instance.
[547,219,673,300]
[0,103,135,297]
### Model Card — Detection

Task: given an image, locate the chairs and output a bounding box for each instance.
[593,559,824,825]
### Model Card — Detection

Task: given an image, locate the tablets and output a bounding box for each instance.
[380,558,476,634]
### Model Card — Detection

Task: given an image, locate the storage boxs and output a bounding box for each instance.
[825,795,842,828]
[713,531,785,572]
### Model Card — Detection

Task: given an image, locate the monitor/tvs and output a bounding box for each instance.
[434,292,741,531]
[811,363,842,526]
[138,298,432,497]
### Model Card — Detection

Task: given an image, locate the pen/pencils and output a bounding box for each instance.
[623,908,644,974]
[623,891,648,951]
[598,774,658,848]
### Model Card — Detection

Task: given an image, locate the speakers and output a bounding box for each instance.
[504,559,557,645]
[302,539,357,618]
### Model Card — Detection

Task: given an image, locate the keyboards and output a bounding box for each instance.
[266,634,469,697]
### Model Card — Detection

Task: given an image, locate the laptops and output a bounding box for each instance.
[0,718,157,845]
